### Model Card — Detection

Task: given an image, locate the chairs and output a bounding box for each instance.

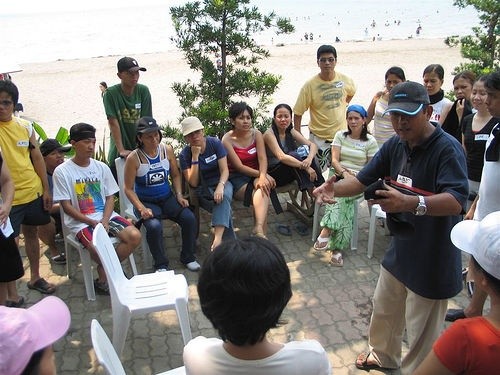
[91,318,186,375]
[366,203,387,259]
[58,201,138,301]
[311,195,372,250]
[114,157,182,270]
[90,222,193,360]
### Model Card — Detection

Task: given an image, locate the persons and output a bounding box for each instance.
[422,63,476,143]
[52,123,142,295]
[272,15,423,43]
[262,104,326,199]
[0,79,71,294]
[177,116,237,252]
[446,70,500,322]
[124,117,200,273]
[293,45,406,174]
[99,56,152,198]
[183,237,339,375]
[314,104,379,266]
[0,295,71,375]
[312,80,471,374]
[413,210,499,375]
[0,152,25,308]
[221,101,276,241]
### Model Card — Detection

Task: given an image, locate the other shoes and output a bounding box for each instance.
[94,279,109,295]
[186,260,201,271]
[249,223,269,241]
[5,295,24,307]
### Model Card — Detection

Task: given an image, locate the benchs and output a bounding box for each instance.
[168,158,317,243]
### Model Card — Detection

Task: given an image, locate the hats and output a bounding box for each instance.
[117,56,147,73]
[381,80,430,119]
[450,210,500,277]
[181,116,204,137]
[135,117,160,134]
[38,138,71,156]
[0,294,71,375]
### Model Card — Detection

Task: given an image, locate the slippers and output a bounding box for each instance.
[279,224,293,236]
[293,221,309,236]
[444,308,467,322]
[313,234,332,250]
[27,278,56,294]
[330,251,343,266]
[47,251,67,265]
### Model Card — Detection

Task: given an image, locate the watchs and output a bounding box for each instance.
[413,196,427,216]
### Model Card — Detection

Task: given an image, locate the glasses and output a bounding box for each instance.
[319,57,336,62]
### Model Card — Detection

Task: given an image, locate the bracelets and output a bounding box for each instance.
[219,181,225,185]
[191,161,199,164]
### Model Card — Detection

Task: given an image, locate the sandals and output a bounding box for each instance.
[354,351,400,373]
